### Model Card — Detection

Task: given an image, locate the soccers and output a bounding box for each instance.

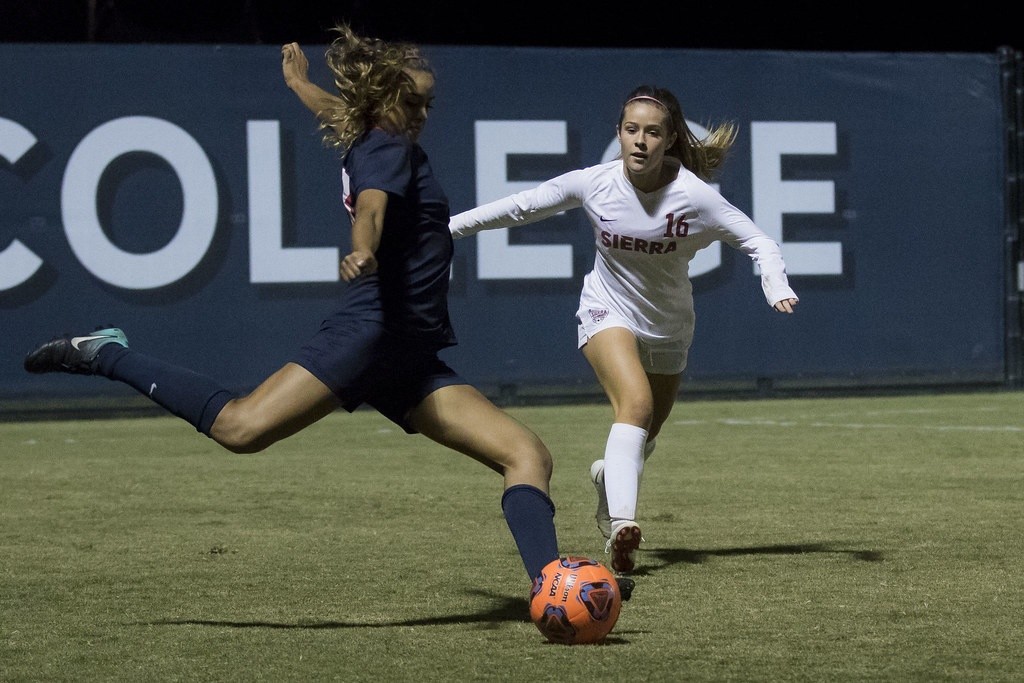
[527,554,622,644]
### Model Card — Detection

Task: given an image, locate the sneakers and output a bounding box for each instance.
[23,324,129,375]
[615,578,635,602]
[590,459,611,539]
[605,521,645,575]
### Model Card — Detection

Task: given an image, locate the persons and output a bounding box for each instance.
[449,84,800,576]
[24,24,637,599]
